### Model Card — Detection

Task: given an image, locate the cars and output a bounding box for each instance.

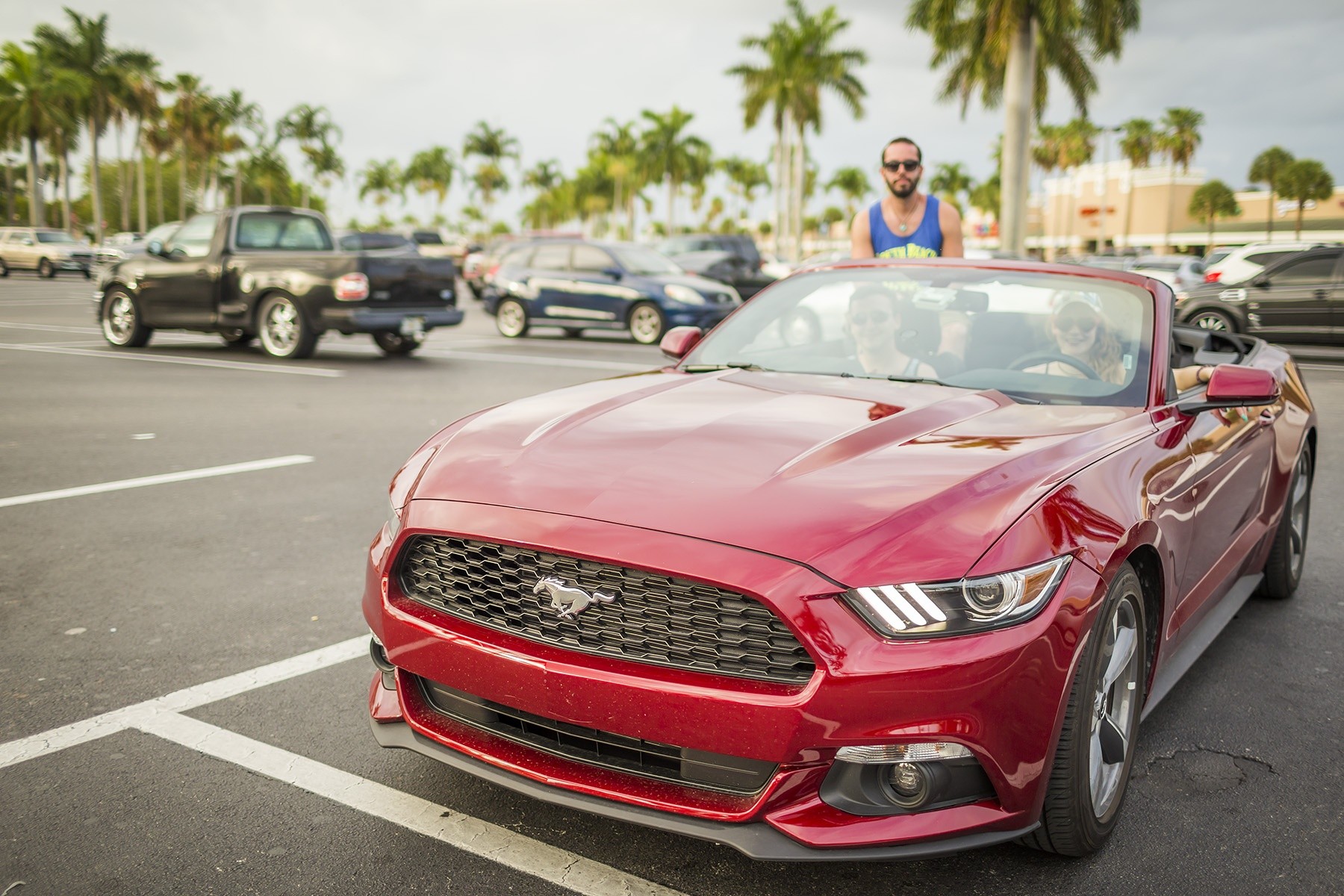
[927,237,1344,373]
[323,228,917,346]
[0,225,144,280]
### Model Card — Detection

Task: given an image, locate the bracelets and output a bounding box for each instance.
[1196,364,1213,384]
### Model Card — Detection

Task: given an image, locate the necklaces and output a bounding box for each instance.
[889,195,919,231]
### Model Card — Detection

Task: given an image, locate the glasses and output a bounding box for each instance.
[845,311,898,322]
[1053,318,1099,333]
[883,159,920,171]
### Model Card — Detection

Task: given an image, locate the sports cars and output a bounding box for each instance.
[359,258,1322,856]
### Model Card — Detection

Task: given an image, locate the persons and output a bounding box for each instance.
[1022,297,1215,391]
[850,138,963,260]
[847,285,938,380]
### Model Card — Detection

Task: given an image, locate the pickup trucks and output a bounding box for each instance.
[86,203,470,361]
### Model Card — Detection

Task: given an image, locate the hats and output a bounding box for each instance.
[1049,289,1103,317]
